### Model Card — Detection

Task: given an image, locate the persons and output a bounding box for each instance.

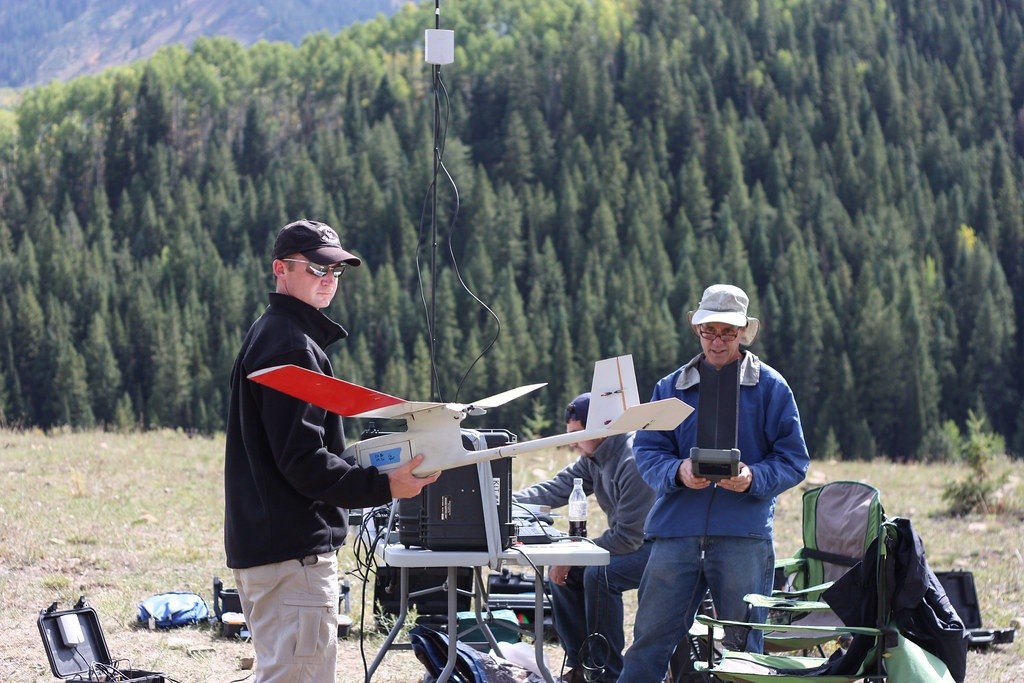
[224,218,441,683]
[512,392,657,683]
[615,284,810,683]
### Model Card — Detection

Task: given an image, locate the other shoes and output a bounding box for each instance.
[558,668,586,683]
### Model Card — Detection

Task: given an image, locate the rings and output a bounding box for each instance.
[734,486,737,490]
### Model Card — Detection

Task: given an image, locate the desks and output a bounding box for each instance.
[361,513,610,683]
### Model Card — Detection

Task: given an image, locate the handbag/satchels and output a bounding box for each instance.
[139,591,208,628]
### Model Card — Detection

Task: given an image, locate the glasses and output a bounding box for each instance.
[699,324,740,341]
[280,258,347,278]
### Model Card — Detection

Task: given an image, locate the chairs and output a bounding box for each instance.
[764,481,882,660]
[694,522,900,683]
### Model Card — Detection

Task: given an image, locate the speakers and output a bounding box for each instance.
[373,566,474,635]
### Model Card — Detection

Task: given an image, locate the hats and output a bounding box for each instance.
[271,219,362,267]
[687,284,760,347]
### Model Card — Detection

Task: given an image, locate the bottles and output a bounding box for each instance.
[568,477,588,542]
